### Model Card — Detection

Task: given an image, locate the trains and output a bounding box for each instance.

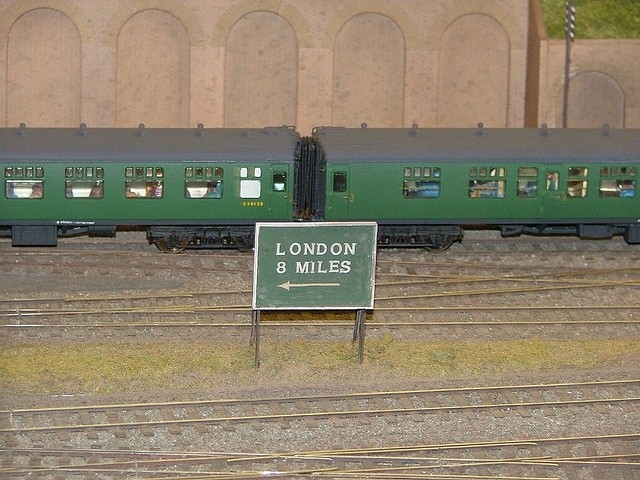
[1,123,640,254]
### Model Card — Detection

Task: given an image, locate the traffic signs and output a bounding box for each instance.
[251,220,378,310]
